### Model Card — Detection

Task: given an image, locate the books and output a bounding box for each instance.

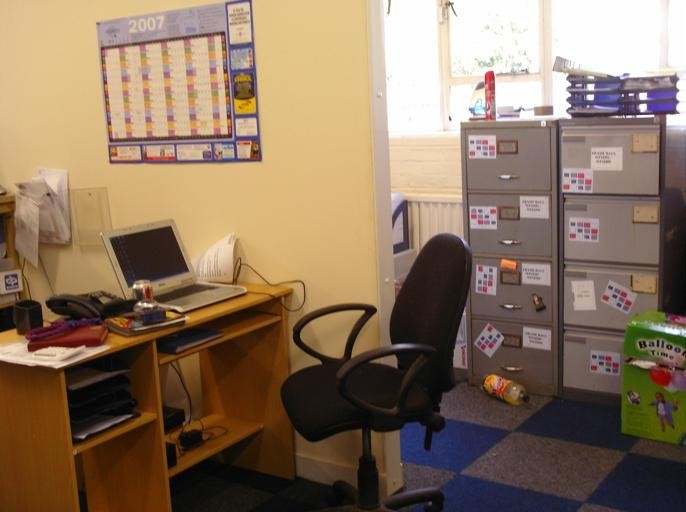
[106,308,186,338]
[26,322,111,348]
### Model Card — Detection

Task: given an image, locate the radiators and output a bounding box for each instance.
[406,196,462,255]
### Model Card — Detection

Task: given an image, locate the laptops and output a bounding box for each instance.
[100,217,248,313]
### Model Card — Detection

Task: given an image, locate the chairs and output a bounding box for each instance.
[281,235,473,510]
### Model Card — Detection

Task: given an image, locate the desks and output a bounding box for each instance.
[0,280,294,512]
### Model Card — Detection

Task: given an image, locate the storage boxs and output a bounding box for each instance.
[621,310,686,446]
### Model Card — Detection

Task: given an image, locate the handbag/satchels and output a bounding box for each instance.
[27,320,109,351]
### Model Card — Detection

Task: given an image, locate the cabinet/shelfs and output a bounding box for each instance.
[463,117,559,395]
[559,112,668,398]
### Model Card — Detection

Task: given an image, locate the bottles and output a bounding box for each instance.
[485,71,496,122]
[481,373,530,407]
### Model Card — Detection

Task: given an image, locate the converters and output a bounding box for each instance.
[177,428,202,449]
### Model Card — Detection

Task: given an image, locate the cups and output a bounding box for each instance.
[14,300,43,335]
